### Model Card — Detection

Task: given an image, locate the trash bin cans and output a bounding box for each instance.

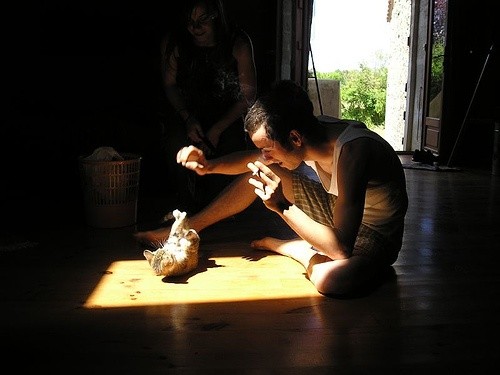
[80,145,141,229]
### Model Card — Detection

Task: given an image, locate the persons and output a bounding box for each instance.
[164,0,258,206]
[132,79,409,300]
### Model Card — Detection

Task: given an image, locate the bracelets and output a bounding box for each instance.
[184,114,192,121]
[178,107,186,112]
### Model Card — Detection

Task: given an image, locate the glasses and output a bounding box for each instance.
[186,13,219,29]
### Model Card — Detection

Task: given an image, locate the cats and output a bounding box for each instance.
[143,209,200,276]
